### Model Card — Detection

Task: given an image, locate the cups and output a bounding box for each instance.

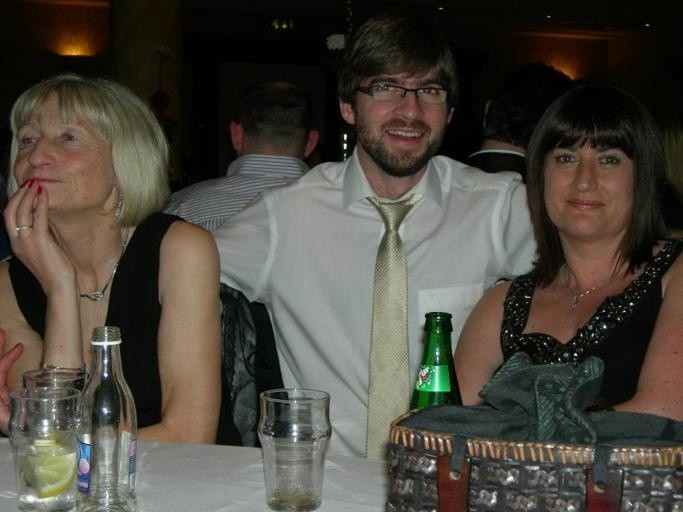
[258,389,333,508]
[9,387,80,508]
[23,368,88,443]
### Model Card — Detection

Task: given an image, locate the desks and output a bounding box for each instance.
[0,436,393,512]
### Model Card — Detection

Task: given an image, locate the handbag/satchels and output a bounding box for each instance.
[382,354,683,511]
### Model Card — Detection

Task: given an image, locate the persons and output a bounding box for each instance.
[466,58,576,175]
[214,7,541,460]
[0,75,221,443]
[452,83,683,423]
[162,79,320,230]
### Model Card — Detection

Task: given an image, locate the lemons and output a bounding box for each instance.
[27,452,78,498]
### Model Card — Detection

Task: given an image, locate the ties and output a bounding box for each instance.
[364,194,422,460]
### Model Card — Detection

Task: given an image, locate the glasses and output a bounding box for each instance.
[357,83,449,105]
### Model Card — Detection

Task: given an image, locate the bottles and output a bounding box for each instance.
[410,312,462,410]
[73,325,137,511]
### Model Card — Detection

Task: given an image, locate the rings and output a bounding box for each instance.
[15,226,31,230]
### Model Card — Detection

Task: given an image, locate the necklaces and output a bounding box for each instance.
[560,263,626,309]
[80,222,130,301]
